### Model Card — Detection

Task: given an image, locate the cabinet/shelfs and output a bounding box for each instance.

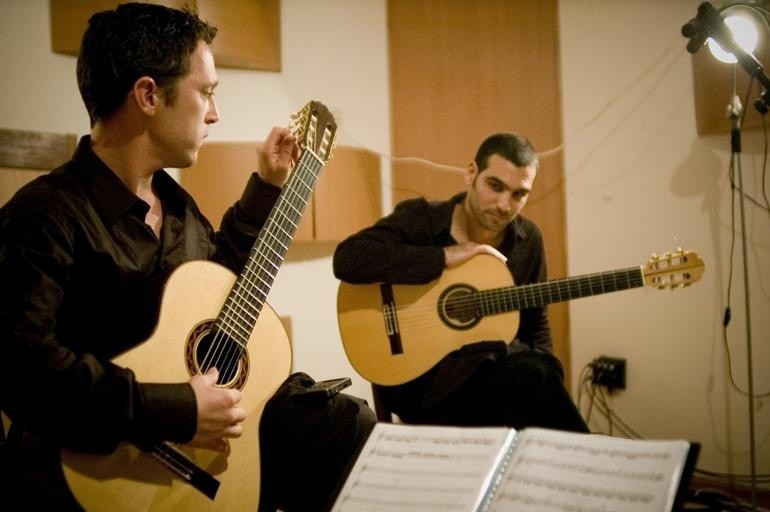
[49,1,282,73]
[179,139,381,260]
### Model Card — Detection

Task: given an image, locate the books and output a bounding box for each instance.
[330,422,701,512]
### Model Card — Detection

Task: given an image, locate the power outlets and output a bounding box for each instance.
[591,356,626,392]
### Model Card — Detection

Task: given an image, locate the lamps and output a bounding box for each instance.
[704,3,761,506]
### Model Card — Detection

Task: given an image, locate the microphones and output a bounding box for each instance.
[686,26,712,53]
[681,17,703,34]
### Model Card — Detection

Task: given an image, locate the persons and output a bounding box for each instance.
[0,1,377,512]
[333,131,590,434]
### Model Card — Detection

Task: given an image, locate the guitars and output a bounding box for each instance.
[60,100,337,511]
[337,247,706,387]
